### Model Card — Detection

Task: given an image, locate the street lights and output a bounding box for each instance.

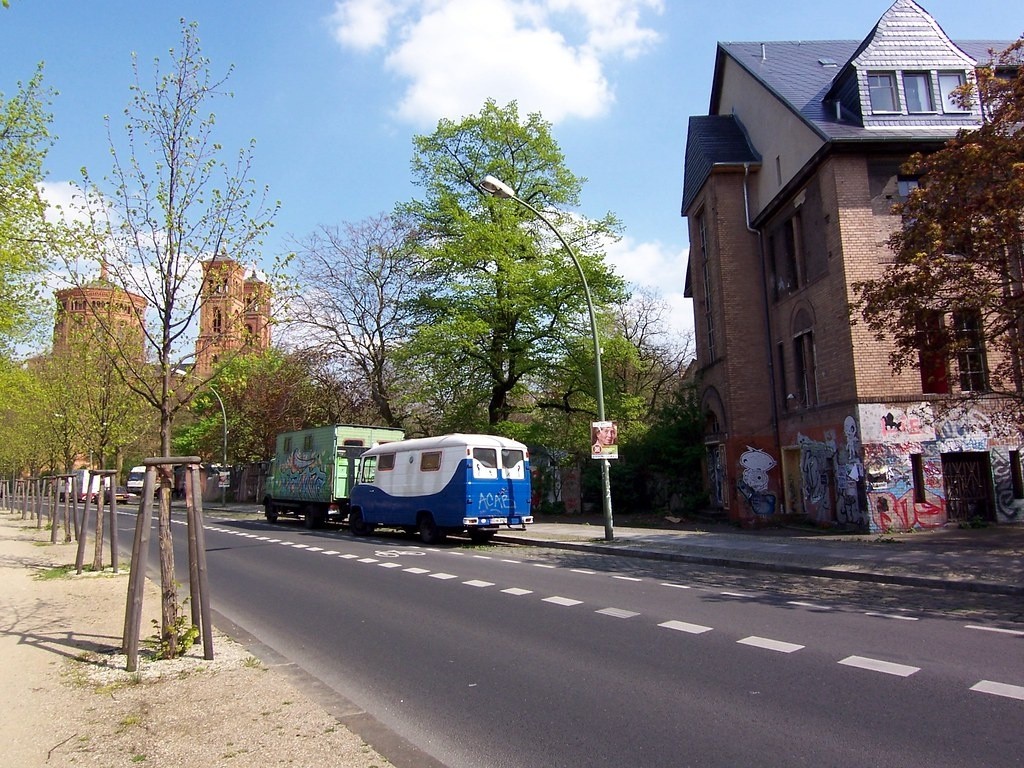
[477,174,616,541]
[170,367,226,508]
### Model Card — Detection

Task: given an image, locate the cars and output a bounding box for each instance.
[93,486,131,505]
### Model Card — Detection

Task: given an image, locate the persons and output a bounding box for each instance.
[219,475,230,485]
[594,425,616,446]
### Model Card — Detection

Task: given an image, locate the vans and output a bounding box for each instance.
[350,431,534,546]
[127,466,176,499]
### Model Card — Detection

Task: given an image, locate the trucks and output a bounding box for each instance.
[54,469,113,503]
[258,424,406,529]
